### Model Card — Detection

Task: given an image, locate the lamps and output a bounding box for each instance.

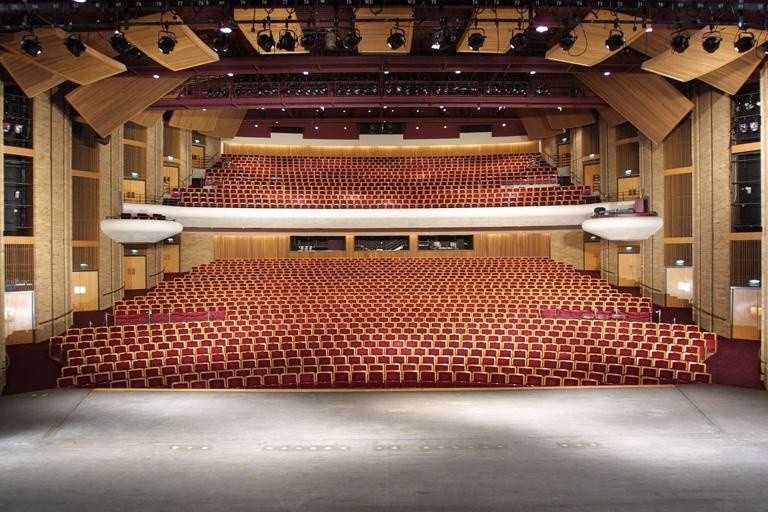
[109,35,132,55]
[158,36,178,54]
[701,36,720,54]
[65,37,87,55]
[257,34,275,51]
[323,34,337,52]
[734,36,756,52]
[671,36,690,53]
[342,32,362,50]
[301,36,317,51]
[468,32,485,52]
[211,33,230,52]
[605,33,624,50]
[558,34,575,49]
[387,32,406,50]
[275,32,297,51]
[20,39,42,55]
[509,33,527,51]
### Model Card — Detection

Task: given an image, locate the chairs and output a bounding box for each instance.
[49,257,717,388]
